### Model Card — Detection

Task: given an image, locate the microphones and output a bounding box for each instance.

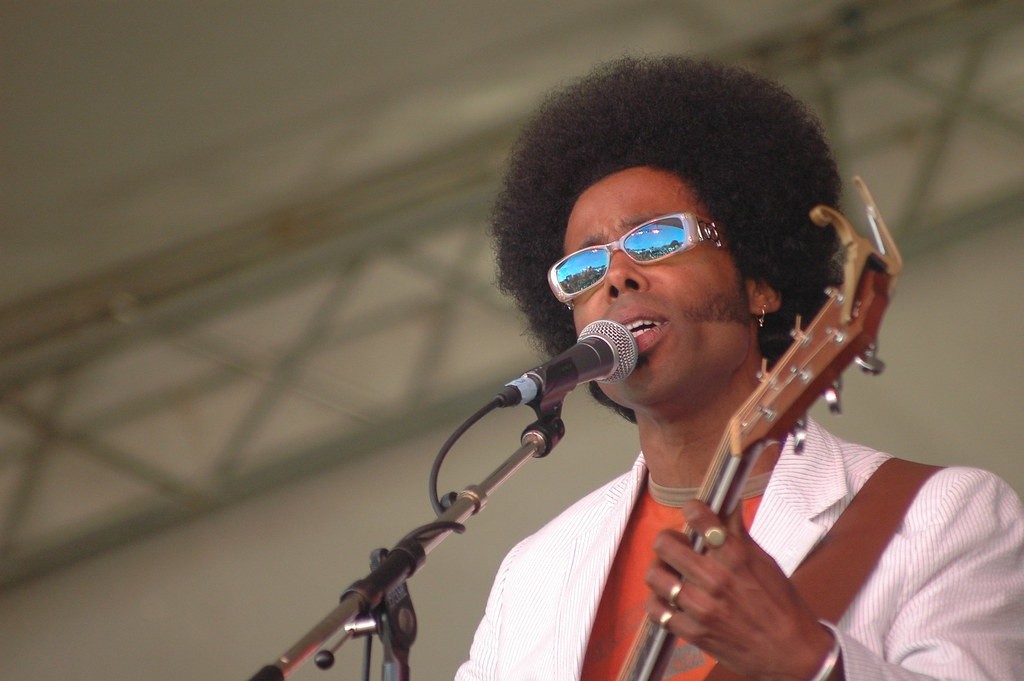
[495,320,638,409]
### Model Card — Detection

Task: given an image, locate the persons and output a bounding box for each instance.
[454,57,1024,681]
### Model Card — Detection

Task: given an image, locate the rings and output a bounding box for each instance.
[668,582,684,607]
[702,526,729,549]
[659,611,678,632]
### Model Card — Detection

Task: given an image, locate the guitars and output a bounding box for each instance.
[614,177,903,681]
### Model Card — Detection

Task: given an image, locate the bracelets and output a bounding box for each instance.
[811,640,841,681]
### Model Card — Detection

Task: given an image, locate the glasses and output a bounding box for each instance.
[547,211,742,311]
